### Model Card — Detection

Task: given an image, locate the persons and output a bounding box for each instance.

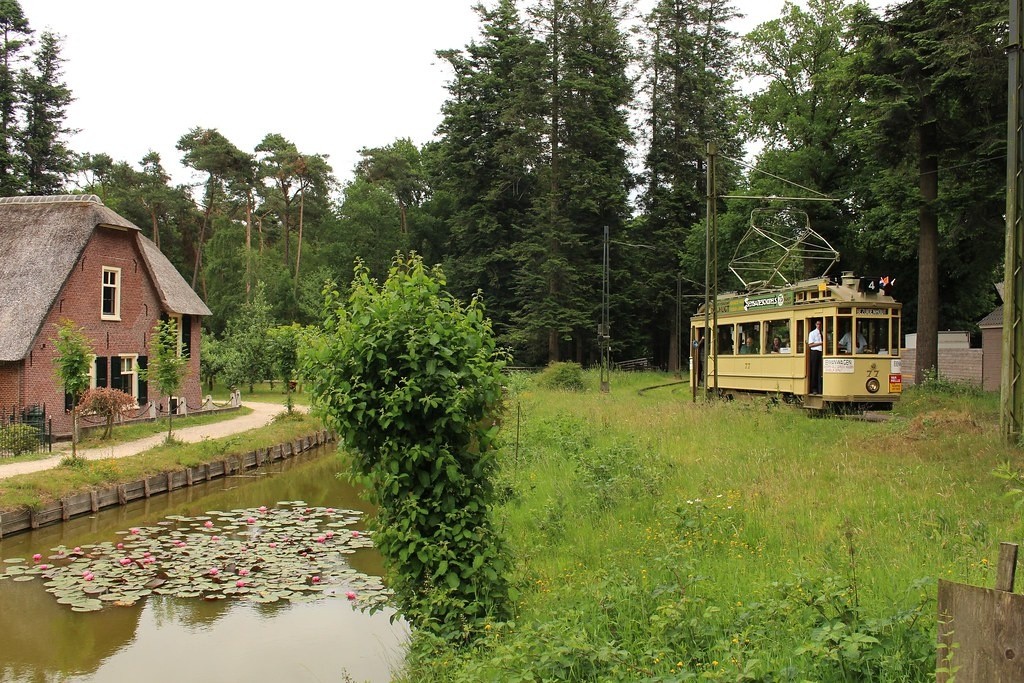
[739,337,757,354]
[838,322,867,354]
[808,319,823,395]
[768,337,786,354]
[718,330,729,355]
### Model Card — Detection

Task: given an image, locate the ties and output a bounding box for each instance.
[856,334,861,349]
[818,331,824,343]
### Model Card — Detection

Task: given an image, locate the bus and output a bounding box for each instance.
[688,270,904,418]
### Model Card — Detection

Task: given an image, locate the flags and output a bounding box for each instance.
[868,275,897,289]
